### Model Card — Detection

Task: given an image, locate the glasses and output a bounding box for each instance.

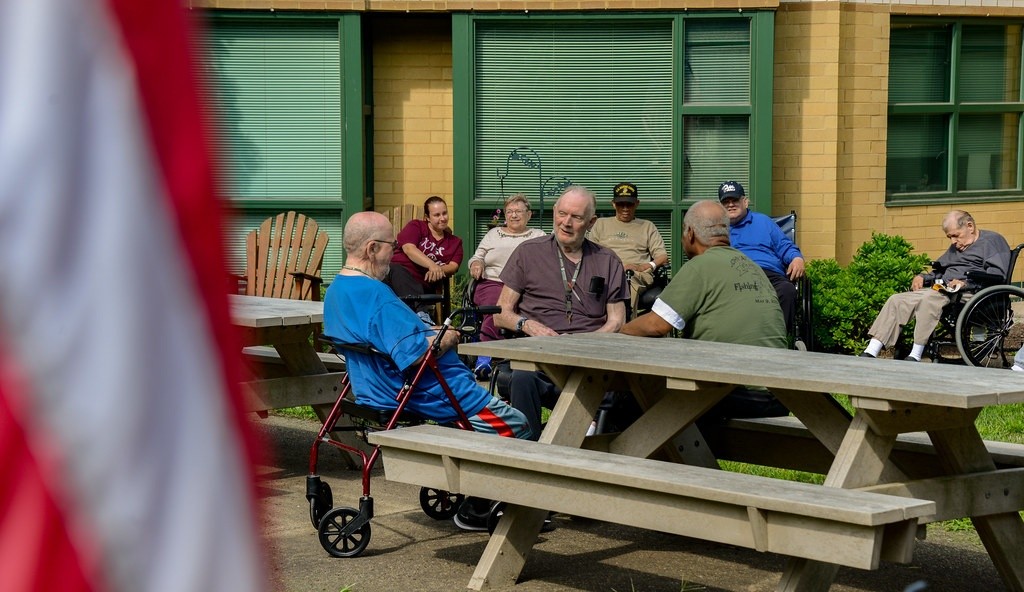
[367,240,399,249]
[505,209,528,215]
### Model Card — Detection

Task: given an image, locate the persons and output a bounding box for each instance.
[323,212,555,530]
[604,199,790,434]
[468,193,547,380]
[718,181,805,331]
[493,186,631,441]
[588,183,667,323]
[860,210,1011,362]
[0,0,274,592]
[383,196,463,328]
[508,227,526,233]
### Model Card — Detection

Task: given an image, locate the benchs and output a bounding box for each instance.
[367,416,1024,570]
[241,346,346,378]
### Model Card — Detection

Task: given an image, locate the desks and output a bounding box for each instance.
[458,332,1024,592]
[229,295,383,470]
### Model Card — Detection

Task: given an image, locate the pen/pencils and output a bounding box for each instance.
[568,282,584,305]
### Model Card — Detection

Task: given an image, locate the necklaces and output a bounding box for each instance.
[343,266,375,279]
[648,262,657,272]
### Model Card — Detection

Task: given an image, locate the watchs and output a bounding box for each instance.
[516,316,528,332]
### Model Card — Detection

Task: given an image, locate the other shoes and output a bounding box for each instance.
[858,351,875,357]
[904,356,918,361]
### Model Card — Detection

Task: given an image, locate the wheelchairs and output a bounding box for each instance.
[456,277,510,381]
[772,209,814,352]
[891,244,1024,369]
[306,295,506,557]
[490,269,634,435]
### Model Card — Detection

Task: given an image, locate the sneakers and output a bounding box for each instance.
[475,362,492,381]
[454,496,554,530]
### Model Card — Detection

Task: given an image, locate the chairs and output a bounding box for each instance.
[228,212,329,352]
[382,204,451,326]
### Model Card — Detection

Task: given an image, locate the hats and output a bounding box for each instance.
[719,181,745,202]
[614,183,637,203]
